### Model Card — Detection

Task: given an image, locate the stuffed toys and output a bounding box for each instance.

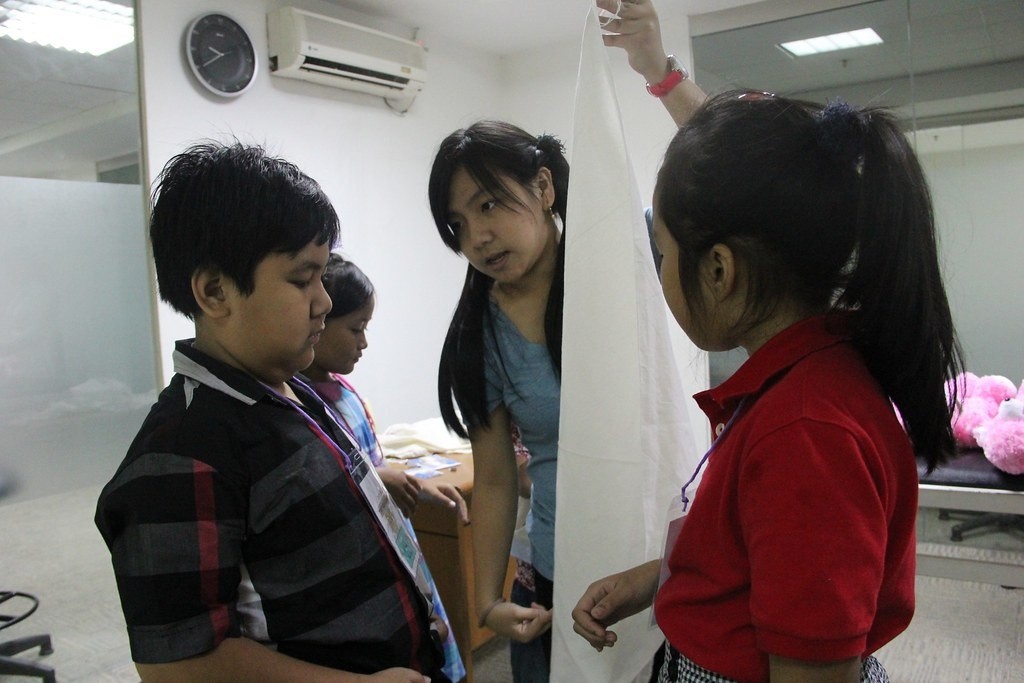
[889,372,1024,476]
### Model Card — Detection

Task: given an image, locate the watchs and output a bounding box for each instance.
[644,53,688,95]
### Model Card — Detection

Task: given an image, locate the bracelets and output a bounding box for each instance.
[476,596,507,627]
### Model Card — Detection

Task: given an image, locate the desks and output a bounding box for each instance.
[380,445,528,683]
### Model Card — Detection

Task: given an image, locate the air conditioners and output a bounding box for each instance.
[267,6,433,100]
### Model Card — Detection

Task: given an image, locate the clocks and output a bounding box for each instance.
[185,11,258,97]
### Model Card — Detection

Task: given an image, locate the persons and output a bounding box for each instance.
[572,87,966,683]
[93,140,449,683]
[425,0,709,682]
[297,253,471,530]
